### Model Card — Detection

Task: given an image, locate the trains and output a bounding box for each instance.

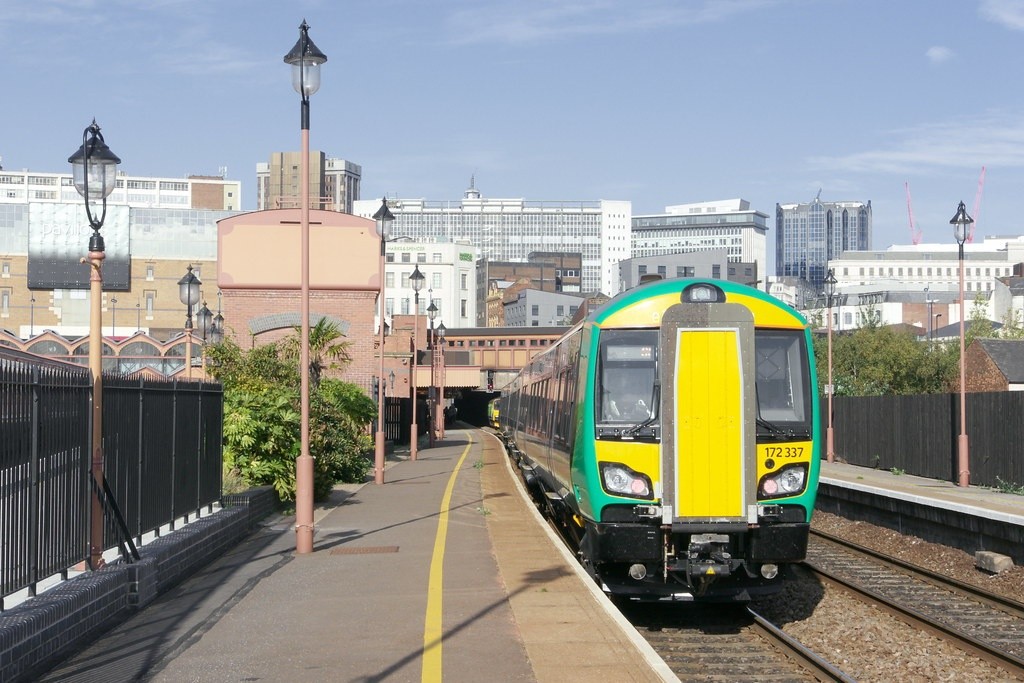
[487,397,500,429]
[498,273,824,614]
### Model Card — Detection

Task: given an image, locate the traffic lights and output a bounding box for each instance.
[488,370,494,390]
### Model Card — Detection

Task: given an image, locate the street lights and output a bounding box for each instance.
[66,117,121,573]
[388,371,396,440]
[408,261,426,461]
[823,267,838,466]
[926,298,942,344]
[176,263,201,381]
[195,300,213,381]
[438,335,447,435]
[436,320,447,441]
[372,195,396,485]
[213,309,225,336]
[948,199,974,488]
[425,298,440,449]
[284,16,329,554]
